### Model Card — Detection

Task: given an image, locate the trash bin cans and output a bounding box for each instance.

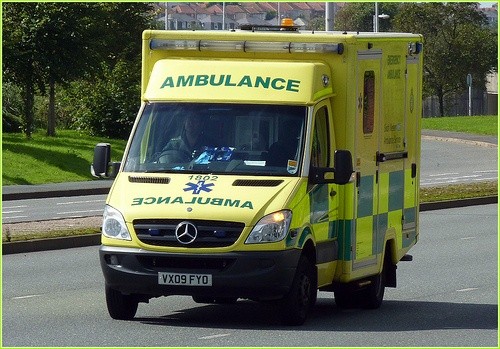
[93,143,121,178]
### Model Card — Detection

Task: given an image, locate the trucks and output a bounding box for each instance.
[92,19,424,327]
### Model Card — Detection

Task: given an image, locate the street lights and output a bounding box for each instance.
[373,13,390,32]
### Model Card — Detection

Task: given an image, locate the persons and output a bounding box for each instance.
[156,111,220,163]
